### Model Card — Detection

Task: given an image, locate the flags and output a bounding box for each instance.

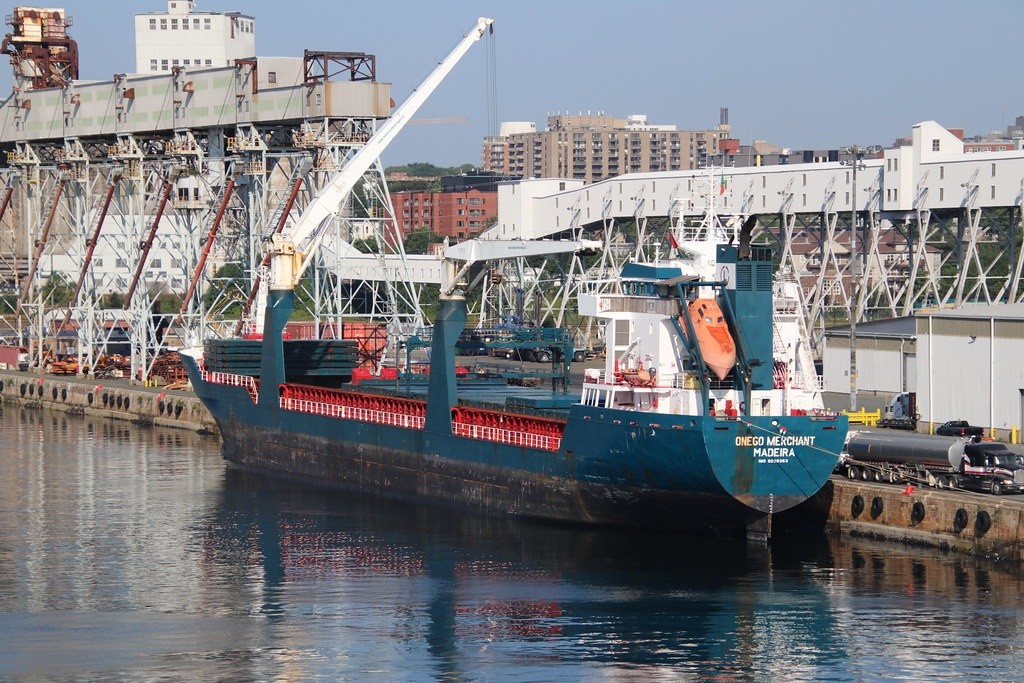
[720,171,725,194]
[669,232,678,249]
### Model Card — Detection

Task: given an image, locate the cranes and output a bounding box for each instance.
[268,14,495,289]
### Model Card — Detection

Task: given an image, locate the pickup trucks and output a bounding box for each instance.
[937,420,985,443]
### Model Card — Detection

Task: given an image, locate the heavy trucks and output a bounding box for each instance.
[844,429,1024,496]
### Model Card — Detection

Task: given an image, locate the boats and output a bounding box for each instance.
[677,298,737,381]
[177,209,851,542]
[620,370,651,387]
